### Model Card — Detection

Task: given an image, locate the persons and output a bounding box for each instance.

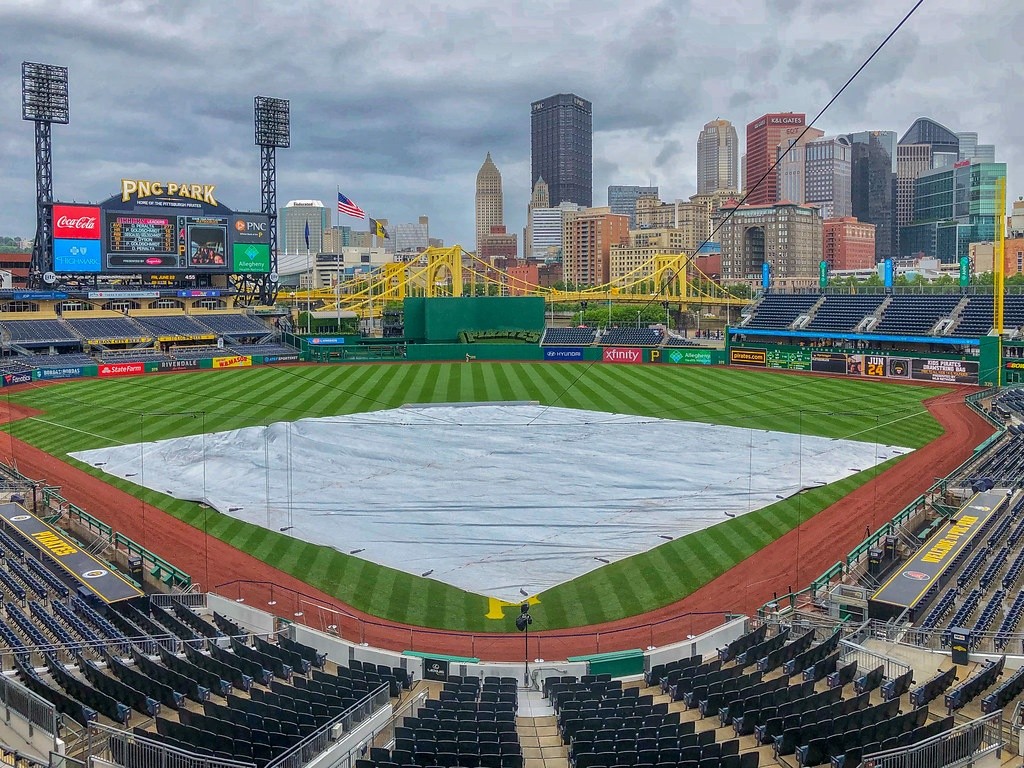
[200,247,224,265]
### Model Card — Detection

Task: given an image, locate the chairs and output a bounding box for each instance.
[0,529,527,768]
[746,294,1024,342]
[543,388,1024,768]
[543,328,709,346]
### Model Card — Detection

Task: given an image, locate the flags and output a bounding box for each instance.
[338,193,365,218]
[370,217,389,238]
[305,220,310,249]
